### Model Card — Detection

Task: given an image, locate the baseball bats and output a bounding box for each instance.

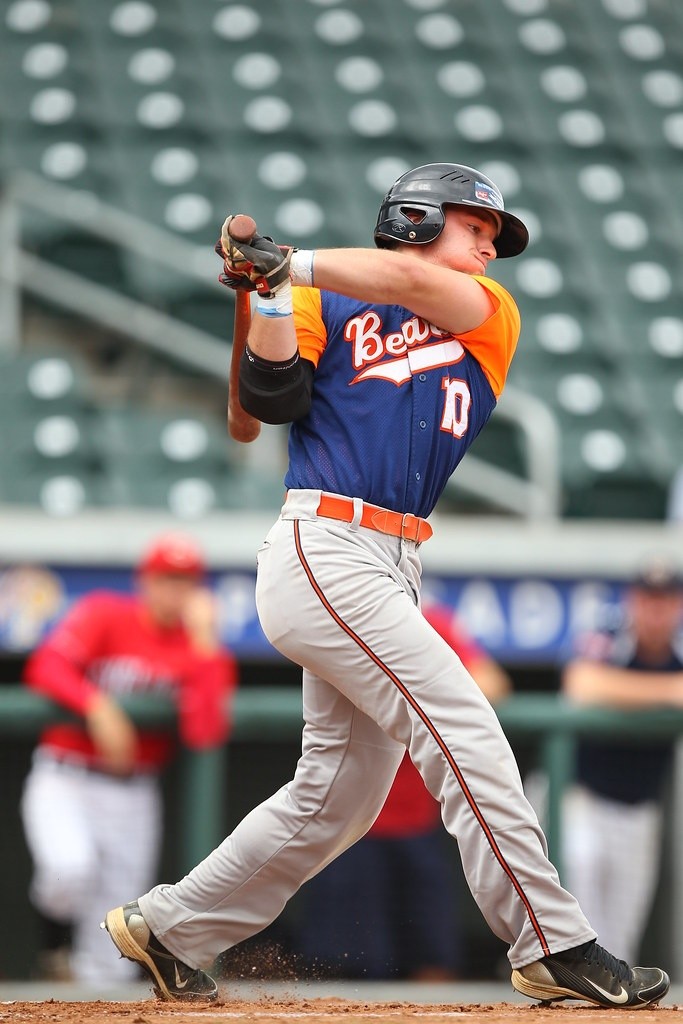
[227,215,262,442]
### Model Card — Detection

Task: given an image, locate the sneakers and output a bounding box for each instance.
[512,940,669,1010]
[106,901,225,1008]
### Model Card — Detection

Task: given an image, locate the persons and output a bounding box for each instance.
[332,601,515,982]
[18,531,235,981]
[555,562,683,968]
[101,162,673,1012]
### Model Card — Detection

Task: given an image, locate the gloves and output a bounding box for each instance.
[215,240,293,291]
[220,214,292,298]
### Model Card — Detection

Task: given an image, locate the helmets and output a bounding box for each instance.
[374,163,528,259]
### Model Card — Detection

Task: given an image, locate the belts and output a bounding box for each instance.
[284,490,434,544]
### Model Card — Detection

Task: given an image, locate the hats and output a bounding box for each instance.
[138,545,207,579]
